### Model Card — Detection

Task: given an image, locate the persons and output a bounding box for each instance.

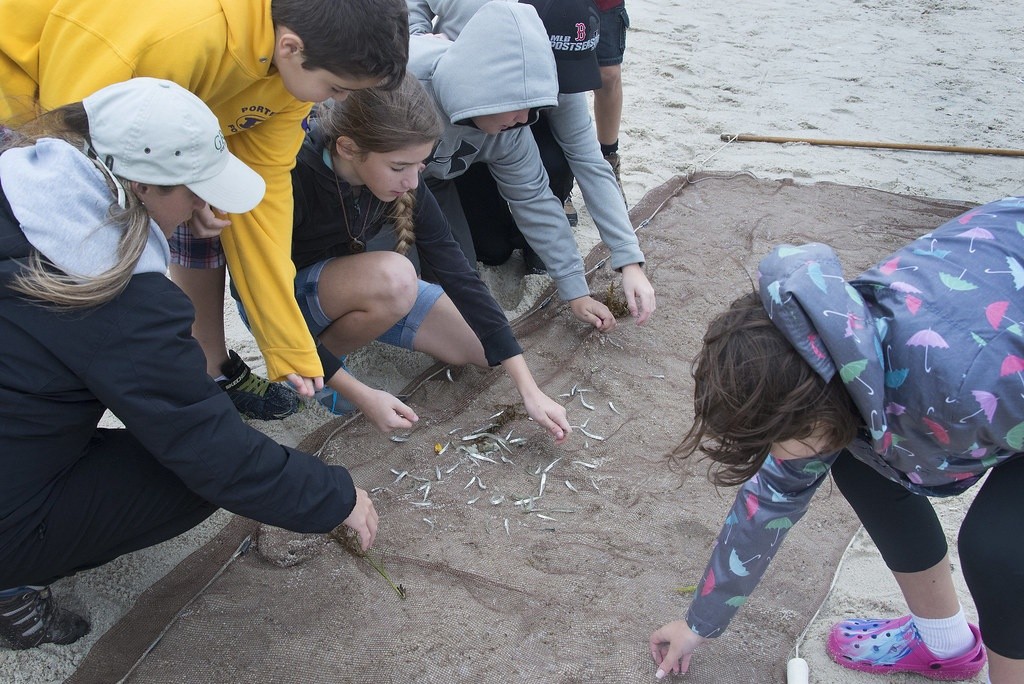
[649,195,1024,684]
[0,0,657,444]
[0,77,377,646]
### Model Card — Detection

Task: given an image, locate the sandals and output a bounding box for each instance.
[824,613,987,681]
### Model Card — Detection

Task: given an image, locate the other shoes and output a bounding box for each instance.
[564,190,579,225]
[605,153,628,212]
[520,238,548,276]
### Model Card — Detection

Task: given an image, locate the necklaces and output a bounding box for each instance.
[330,150,374,254]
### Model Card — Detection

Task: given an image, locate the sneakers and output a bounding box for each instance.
[214,349,300,422]
[0,585,90,650]
[281,353,361,416]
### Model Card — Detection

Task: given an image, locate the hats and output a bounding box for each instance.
[81,76,268,214]
[518,0,602,94]
